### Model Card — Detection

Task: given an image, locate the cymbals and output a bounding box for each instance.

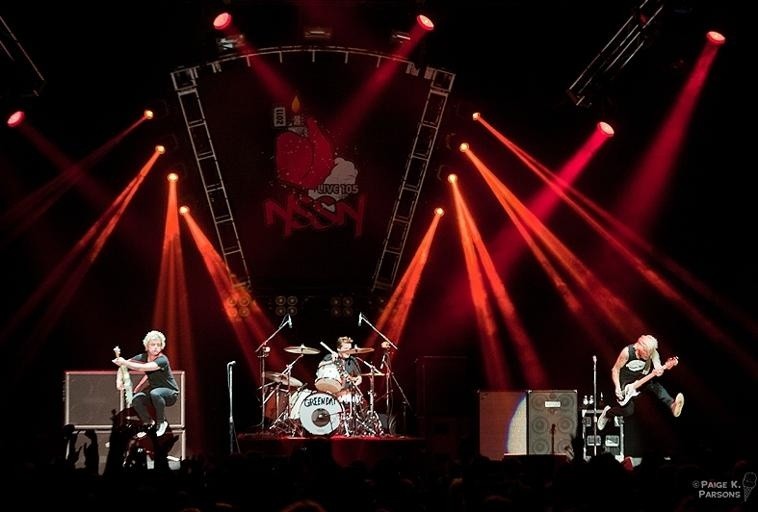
[264,372,303,386]
[285,346,320,354]
[341,348,374,355]
[361,373,385,377]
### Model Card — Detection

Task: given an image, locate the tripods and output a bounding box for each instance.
[261,388,301,437]
[343,382,386,436]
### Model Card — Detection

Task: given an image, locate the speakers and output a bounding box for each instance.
[65,370,124,429]
[477,388,577,461]
[503,455,570,468]
[127,369,186,430]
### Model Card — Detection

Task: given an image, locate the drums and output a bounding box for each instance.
[300,392,345,435]
[265,388,290,420]
[316,364,341,394]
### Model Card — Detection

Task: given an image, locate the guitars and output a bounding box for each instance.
[617,356,680,408]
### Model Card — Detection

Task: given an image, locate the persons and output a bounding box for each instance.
[597,335,684,431]
[0,422,758,512]
[322,336,365,429]
[113,330,180,438]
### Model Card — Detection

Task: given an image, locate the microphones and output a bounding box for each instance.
[592,355,597,364]
[358,312,362,327]
[288,314,292,328]
[379,354,385,369]
[228,360,236,366]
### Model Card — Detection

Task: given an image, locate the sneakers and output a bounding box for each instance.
[597,405,612,431]
[156,420,168,437]
[671,392,685,418]
[136,419,155,438]
